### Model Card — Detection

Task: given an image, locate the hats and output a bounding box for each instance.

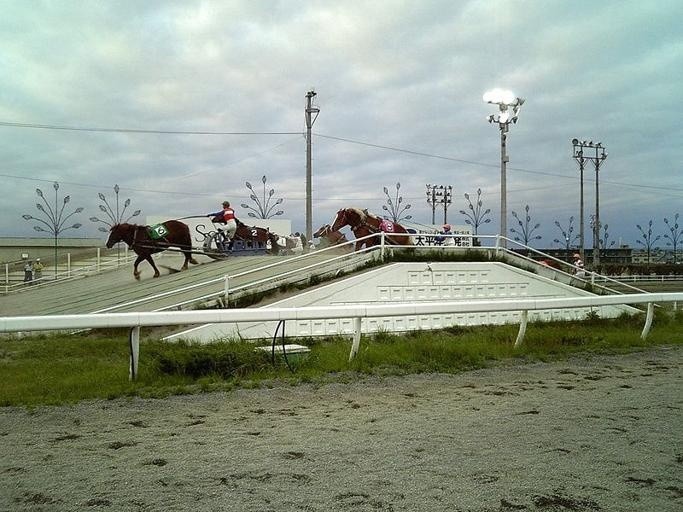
[220,200,230,206]
[442,223,451,230]
[573,254,581,259]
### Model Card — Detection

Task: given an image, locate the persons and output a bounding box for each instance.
[288,232,304,253]
[24,259,33,285]
[441,224,456,247]
[212,201,238,250]
[32,258,43,279]
[571,253,586,277]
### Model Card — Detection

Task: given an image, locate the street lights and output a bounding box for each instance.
[19,180,84,279]
[635,216,662,264]
[424,183,452,226]
[664,212,683,267]
[304,90,322,248]
[380,180,412,224]
[588,214,602,263]
[550,216,582,262]
[240,175,284,219]
[589,221,617,262]
[483,86,526,254]
[87,182,143,251]
[511,205,542,255]
[459,187,491,246]
[569,135,606,252]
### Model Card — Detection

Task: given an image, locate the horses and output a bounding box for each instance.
[313,224,354,246]
[330,207,415,254]
[212,209,280,255]
[106,219,199,281]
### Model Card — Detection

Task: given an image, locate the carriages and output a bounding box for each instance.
[105,220,267,282]
